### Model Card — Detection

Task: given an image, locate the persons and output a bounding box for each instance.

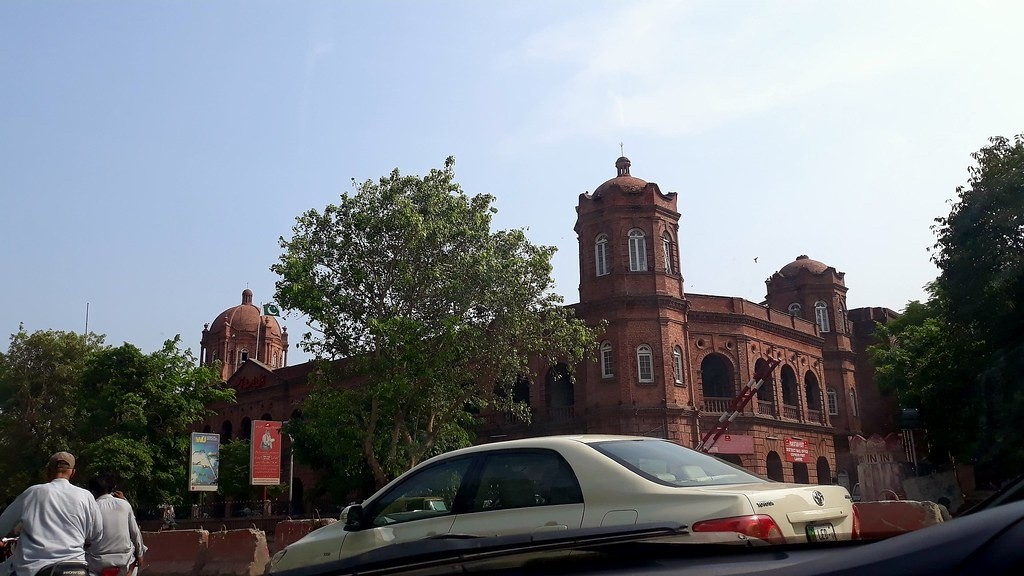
[87,475,147,576]
[0,452,103,576]
[938,497,953,520]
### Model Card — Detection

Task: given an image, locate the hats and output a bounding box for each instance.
[45,451,75,469]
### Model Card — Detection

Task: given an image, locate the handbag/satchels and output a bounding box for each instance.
[101,566,128,576]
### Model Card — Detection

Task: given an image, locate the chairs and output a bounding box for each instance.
[488,467,535,507]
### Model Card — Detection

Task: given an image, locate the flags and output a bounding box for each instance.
[264,305,280,316]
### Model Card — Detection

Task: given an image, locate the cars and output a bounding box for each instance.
[264,435,862,576]
